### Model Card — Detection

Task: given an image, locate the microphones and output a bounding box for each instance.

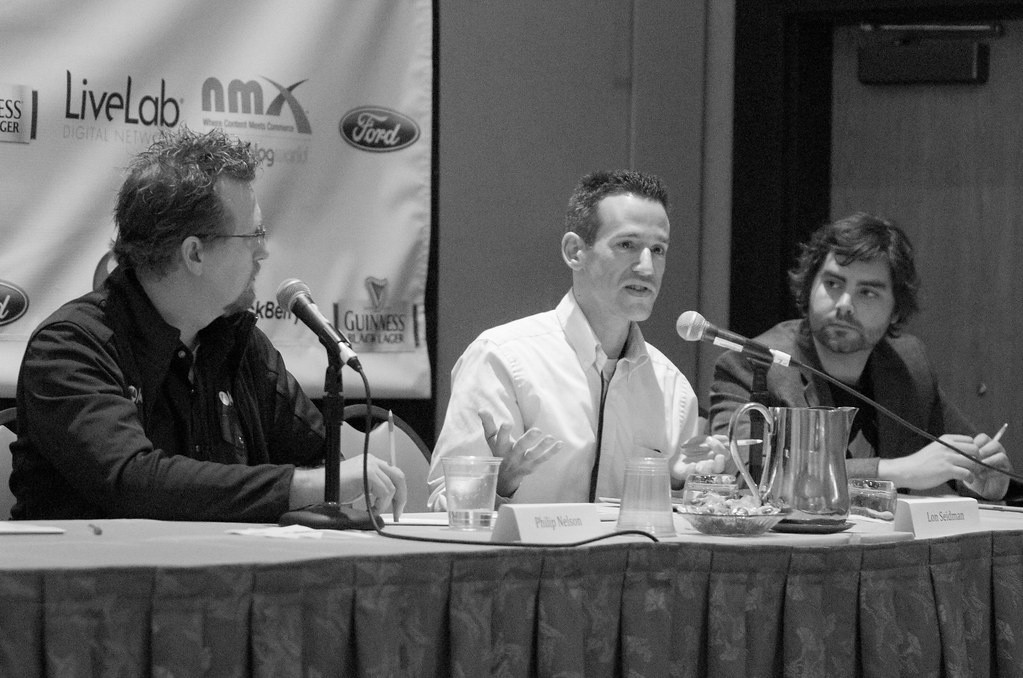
[274,277,362,373]
[675,311,804,370]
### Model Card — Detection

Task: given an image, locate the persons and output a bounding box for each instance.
[9,124,407,525]
[426,162,732,512]
[708,211,1023,502]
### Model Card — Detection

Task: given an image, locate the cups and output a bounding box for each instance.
[614,458,679,539]
[441,455,503,531]
[683,472,740,507]
[846,476,899,522]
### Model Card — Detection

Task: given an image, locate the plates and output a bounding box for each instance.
[673,504,784,537]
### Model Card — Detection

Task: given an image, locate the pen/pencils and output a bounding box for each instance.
[680,439,763,449]
[992,423,1008,441]
[388,409,401,523]
[85,523,102,535]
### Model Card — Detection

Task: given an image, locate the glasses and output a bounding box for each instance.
[194,225,266,242]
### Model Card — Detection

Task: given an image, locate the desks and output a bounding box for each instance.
[0,500,1023,678]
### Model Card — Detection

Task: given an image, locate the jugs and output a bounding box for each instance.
[728,402,860,534]
